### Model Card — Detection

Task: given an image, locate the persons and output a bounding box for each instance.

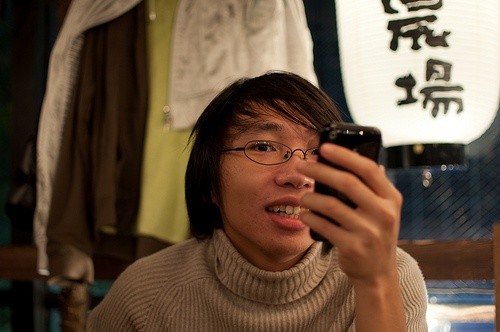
[84,69,430,332]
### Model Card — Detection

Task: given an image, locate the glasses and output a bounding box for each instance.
[218,140,321,165]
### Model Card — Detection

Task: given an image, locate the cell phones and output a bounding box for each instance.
[309,122,381,241]
